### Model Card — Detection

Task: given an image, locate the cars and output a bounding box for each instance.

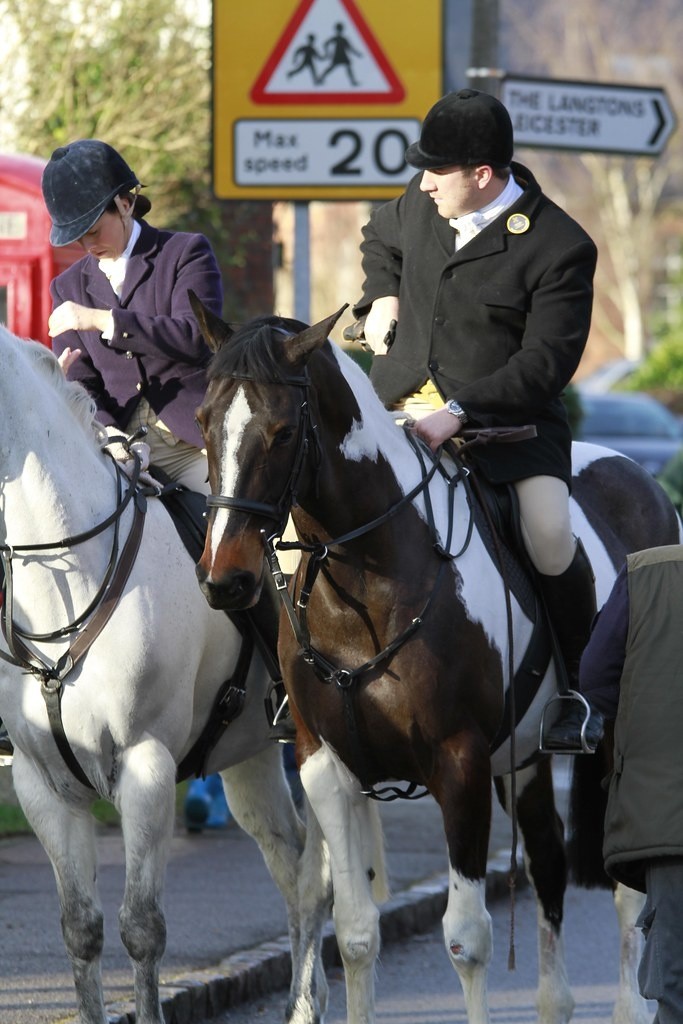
[567,391,683,485]
[570,358,653,401]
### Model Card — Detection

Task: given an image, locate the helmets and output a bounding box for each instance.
[41,140,139,248]
[405,89,514,171]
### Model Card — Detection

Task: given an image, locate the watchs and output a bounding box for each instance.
[446,399,467,424]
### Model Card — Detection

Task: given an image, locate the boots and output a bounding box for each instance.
[246,565,307,742]
[529,533,605,748]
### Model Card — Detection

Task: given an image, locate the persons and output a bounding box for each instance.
[42,139,289,712]
[580,544,683,1024]
[343,89,605,751]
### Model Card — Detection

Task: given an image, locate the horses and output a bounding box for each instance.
[0,323,392,1024]
[187,285,683,1024]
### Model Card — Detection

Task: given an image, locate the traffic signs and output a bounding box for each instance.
[497,71,681,163]
